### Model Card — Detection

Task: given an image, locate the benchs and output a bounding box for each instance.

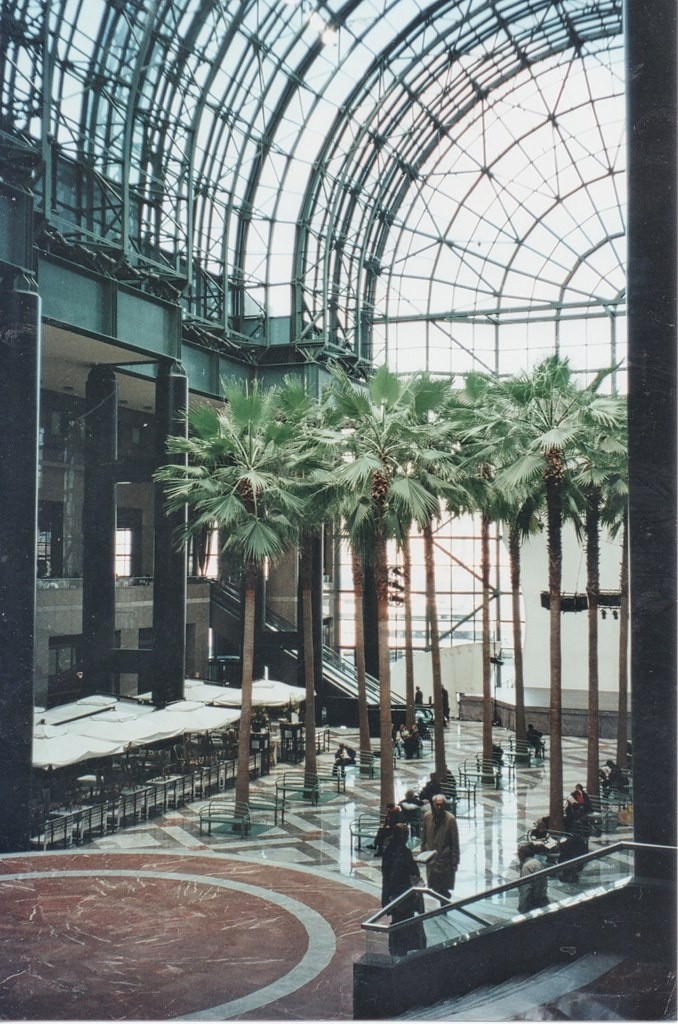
[26,716,633,879]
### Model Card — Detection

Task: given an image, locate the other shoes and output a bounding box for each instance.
[372,851,381,857]
[366,844,377,850]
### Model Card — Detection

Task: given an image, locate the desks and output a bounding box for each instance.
[49,804,94,849]
[76,775,107,798]
[145,775,184,811]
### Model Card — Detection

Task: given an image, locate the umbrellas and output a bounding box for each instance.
[31,679,317,771]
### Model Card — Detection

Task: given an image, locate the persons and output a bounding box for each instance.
[567,784,592,817]
[420,794,459,916]
[367,764,457,857]
[601,740,632,798]
[493,743,505,766]
[442,685,449,726]
[415,686,423,705]
[228,727,238,749]
[331,742,356,777]
[391,718,431,758]
[527,724,545,758]
[380,824,428,956]
[511,841,551,913]
